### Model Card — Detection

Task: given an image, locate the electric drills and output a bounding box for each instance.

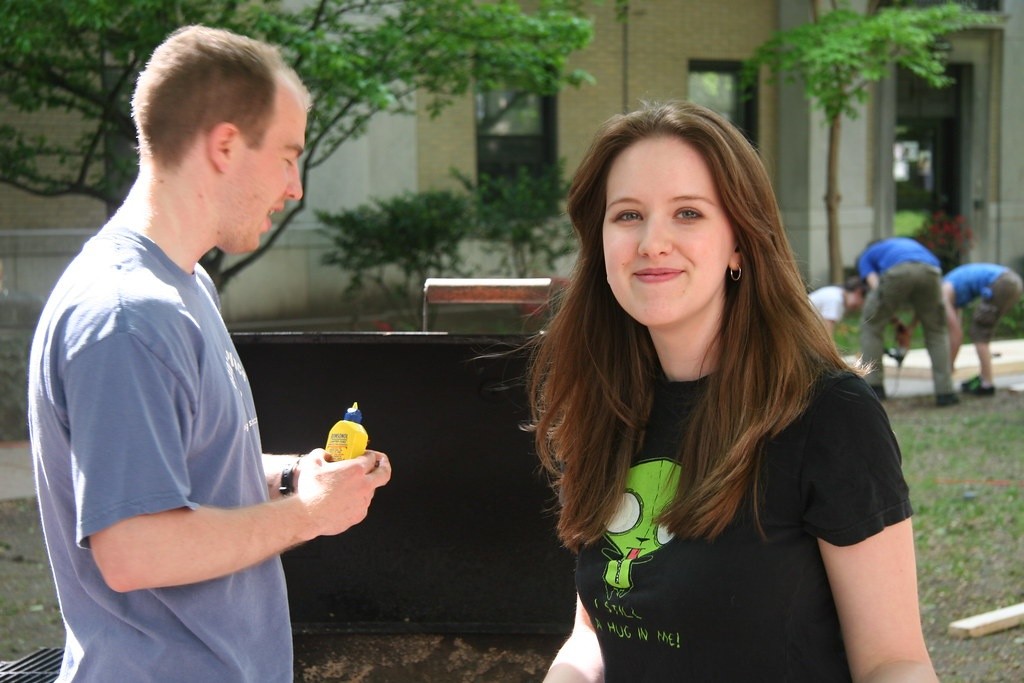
[882,324,911,397]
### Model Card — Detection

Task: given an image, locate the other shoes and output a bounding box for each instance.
[962,378,995,395]
[871,384,886,400]
[937,393,959,406]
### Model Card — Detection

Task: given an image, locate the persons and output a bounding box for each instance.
[805,235,1024,406]
[541,103,944,683]
[25,23,391,682]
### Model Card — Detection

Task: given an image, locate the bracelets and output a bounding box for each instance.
[279,453,301,497]
